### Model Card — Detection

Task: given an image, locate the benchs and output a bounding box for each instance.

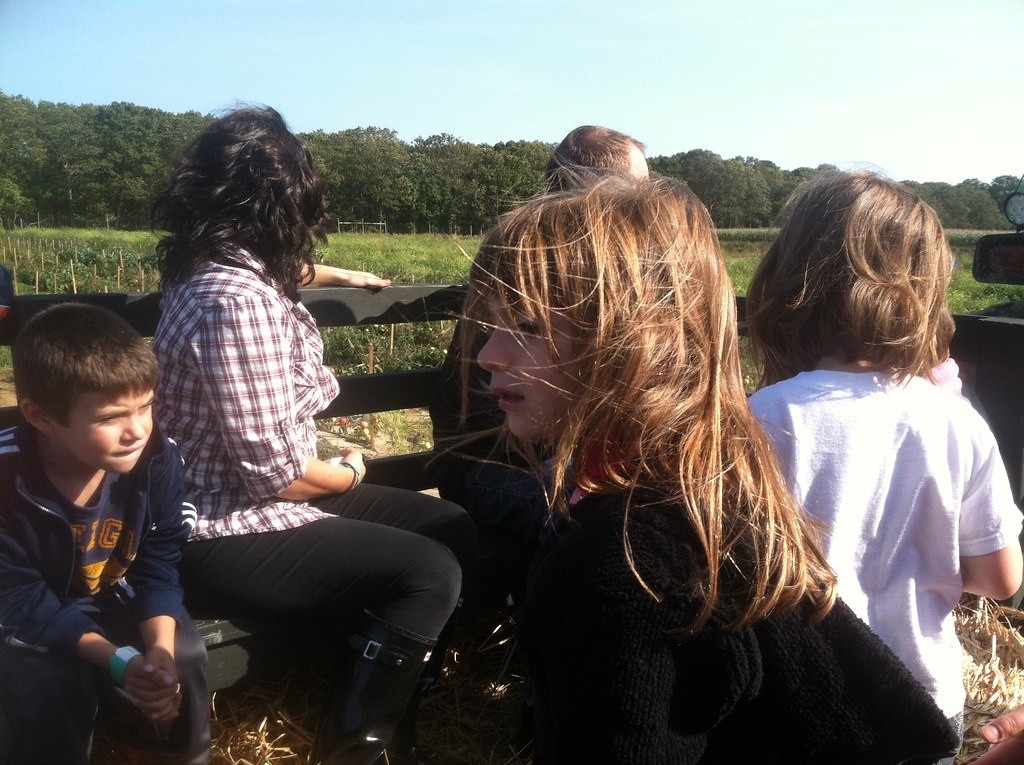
[0,286,531,720]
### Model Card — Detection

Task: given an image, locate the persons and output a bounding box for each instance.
[738,176,1023,741]
[443,173,959,765]
[146,103,493,765]
[430,121,668,593]
[962,704,1024,765]
[1,304,218,764]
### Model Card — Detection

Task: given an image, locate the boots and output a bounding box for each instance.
[377,598,464,765]
[307,608,439,765]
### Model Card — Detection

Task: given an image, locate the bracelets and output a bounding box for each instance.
[338,462,361,495]
[108,644,142,685]
